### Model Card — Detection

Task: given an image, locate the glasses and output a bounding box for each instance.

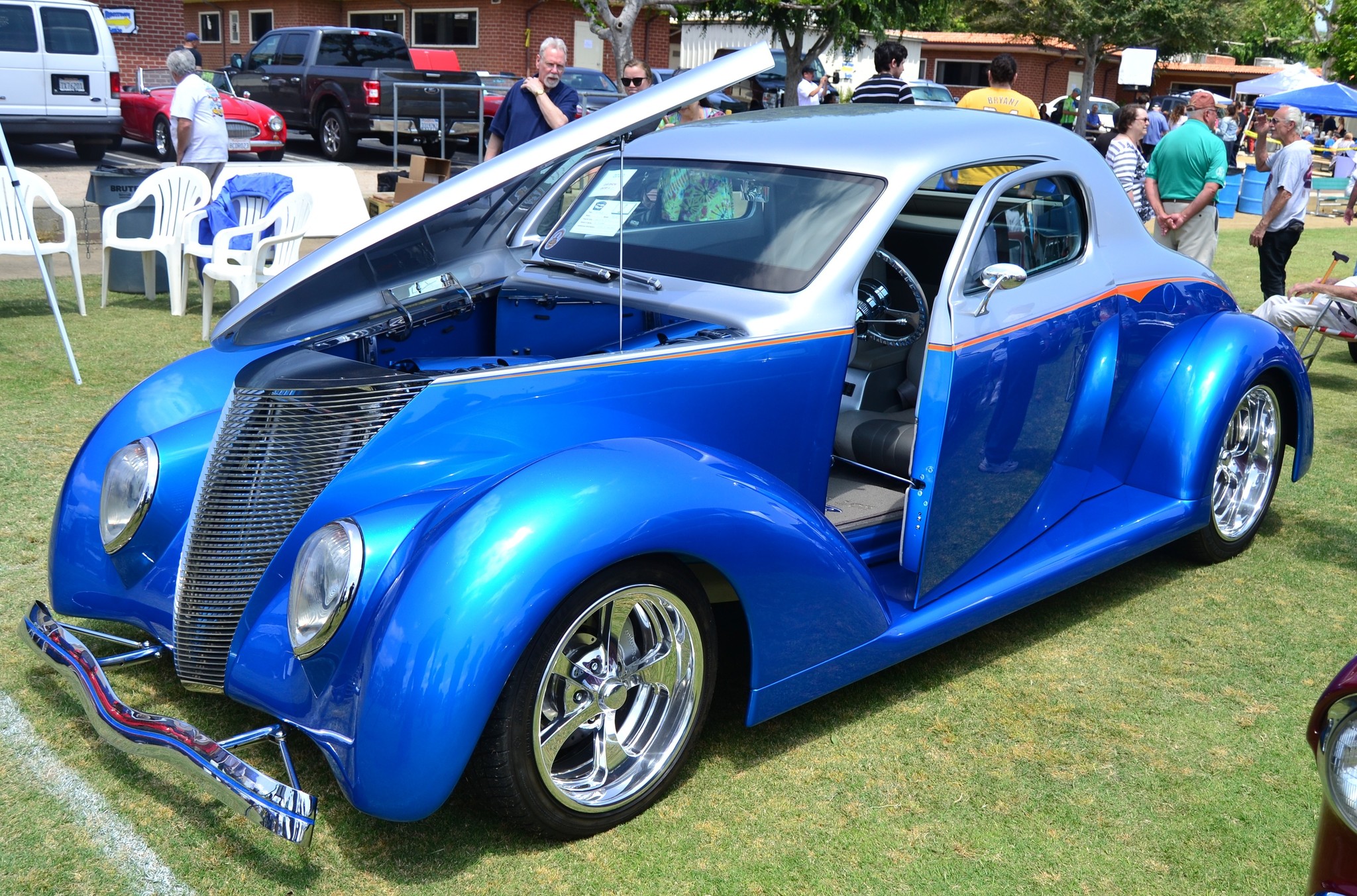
[1134,117,1150,122]
[621,77,648,87]
[1269,118,1287,124]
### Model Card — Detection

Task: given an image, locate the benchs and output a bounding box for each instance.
[1307,176,1357,219]
[1312,154,1331,172]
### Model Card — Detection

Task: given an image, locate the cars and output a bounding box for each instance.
[1149,94,1189,113]
[407,49,506,154]
[906,78,960,107]
[1037,95,1120,132]
[648,68,747,114]
[560,67,621,112]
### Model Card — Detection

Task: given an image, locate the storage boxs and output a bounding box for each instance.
[367,154,452,218]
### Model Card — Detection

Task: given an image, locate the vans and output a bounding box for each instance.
[0,0,125,166]
[713,47,841,113]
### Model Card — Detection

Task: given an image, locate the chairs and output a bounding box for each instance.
[0,164,84,317]
[177,167,292,315]
[571,79,580,87]
[1295,293,1357,374]
[195,189,316,342]
[100,165,213,314]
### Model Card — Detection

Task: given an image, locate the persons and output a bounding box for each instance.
[1212,98,1264,169]
[655,67,734,223]
[1254,104,1313,304]
[1250,276,1357,346]
[484,36,579,237]
[943,55,1040,199]
[1105,103,1153,225]
[622,59,653,96]
[1039,89,1189,161]
[851,42,915,106]
[797,65,829,108]
[167,49,228,207]
[1302,112,1357,225]
[175,32,202,76]
[1144,91,1228,270]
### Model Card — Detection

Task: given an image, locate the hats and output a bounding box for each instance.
[802,65,818,73]
[1334,133,1340,138]
[186,32,202,41]
[1185,91,1228,111]
[1073,88,1081,95]
[1303,126,1312,134]
[1151,102,1162,107]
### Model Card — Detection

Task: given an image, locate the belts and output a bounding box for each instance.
[1162,199,1216,207]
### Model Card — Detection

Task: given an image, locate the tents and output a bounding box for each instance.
[1239,81,1357,154]
[1233,62,1328,101]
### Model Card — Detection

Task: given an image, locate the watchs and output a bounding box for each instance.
[534,89,545,98]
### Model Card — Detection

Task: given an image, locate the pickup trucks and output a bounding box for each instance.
[211,26,487,164]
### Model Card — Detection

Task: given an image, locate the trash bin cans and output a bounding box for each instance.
[91,166,169,294]
[1238,164,1269,216]
[1217,166,1241,218]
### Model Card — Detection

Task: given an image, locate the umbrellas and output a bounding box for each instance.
[1171,88,1233,105]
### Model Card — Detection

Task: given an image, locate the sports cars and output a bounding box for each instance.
[22,23,1316,846]
[121,66,287,162]
